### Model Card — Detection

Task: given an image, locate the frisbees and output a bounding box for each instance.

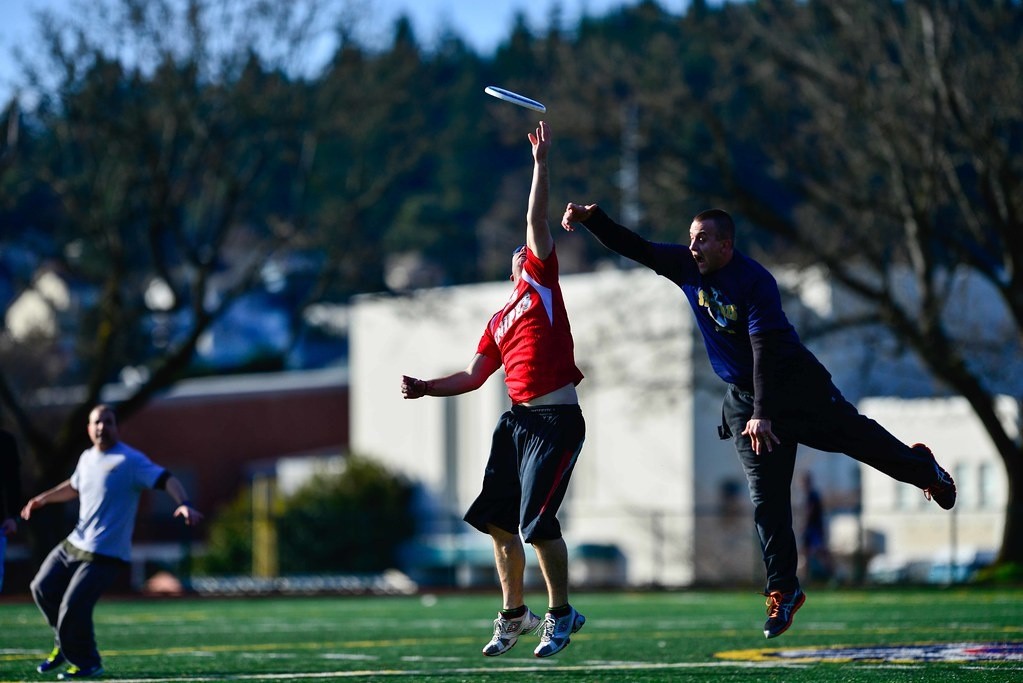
[484,84,548,115]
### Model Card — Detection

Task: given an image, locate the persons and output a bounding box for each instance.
[401,120,587,658]
[797,470,826,588]
[21,405,204,680]
[561,200,957,639]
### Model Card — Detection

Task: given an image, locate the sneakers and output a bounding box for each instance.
[37,645,66,674]
[912,442,956,510]
[482,604,541,657]
[533,604,585,658]
[754,586,806,639]
[57,664,104,681]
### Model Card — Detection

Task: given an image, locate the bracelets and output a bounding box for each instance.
[181,501,193,510]
